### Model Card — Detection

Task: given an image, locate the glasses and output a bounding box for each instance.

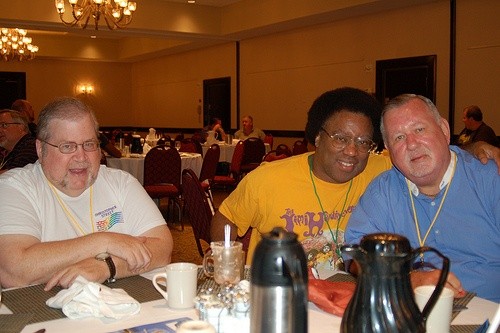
[320,127,377,154]
[0,122,27,129]
[38,139,100,154]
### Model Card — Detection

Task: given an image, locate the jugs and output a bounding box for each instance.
[339,233,450,333]
[250,226,307,333]
[206,130,218,144]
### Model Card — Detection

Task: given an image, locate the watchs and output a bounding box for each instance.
[96,253,116,282]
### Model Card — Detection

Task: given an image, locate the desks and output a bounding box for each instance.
[201,138,271,178]
[106,152,203,205]
[139,138,174,150]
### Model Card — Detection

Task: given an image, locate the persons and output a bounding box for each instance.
[0,99,38,175]
[211,115,265,178]
[344,93,500,305]
[211,85,500,269]
[457,105,495,148]
[0,97,173,291]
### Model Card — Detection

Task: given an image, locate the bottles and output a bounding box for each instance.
[195,282,250,333]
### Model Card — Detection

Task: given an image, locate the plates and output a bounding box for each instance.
[108,302,141,316]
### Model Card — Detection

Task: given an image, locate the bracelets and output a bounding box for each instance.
[219,130,223,133]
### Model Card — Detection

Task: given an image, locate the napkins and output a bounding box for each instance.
[139,266,167,288]
[45,275,141,325]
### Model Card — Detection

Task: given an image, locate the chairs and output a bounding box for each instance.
[100,129,307,256]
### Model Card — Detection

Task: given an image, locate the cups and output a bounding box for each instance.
[164,142,170,150]
[225,135,232,145]
[152,262,198,310]
[241,251,246,280]
[413,286,454,333]
[114,143,130,158]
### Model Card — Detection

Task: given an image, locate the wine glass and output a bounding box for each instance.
[203,240,243,301]
[175,141,181,153]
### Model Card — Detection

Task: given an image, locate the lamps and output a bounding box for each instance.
[0,28,39,62]
[55,0,136,32]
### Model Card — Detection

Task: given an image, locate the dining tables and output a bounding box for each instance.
[0,267,500,333]
